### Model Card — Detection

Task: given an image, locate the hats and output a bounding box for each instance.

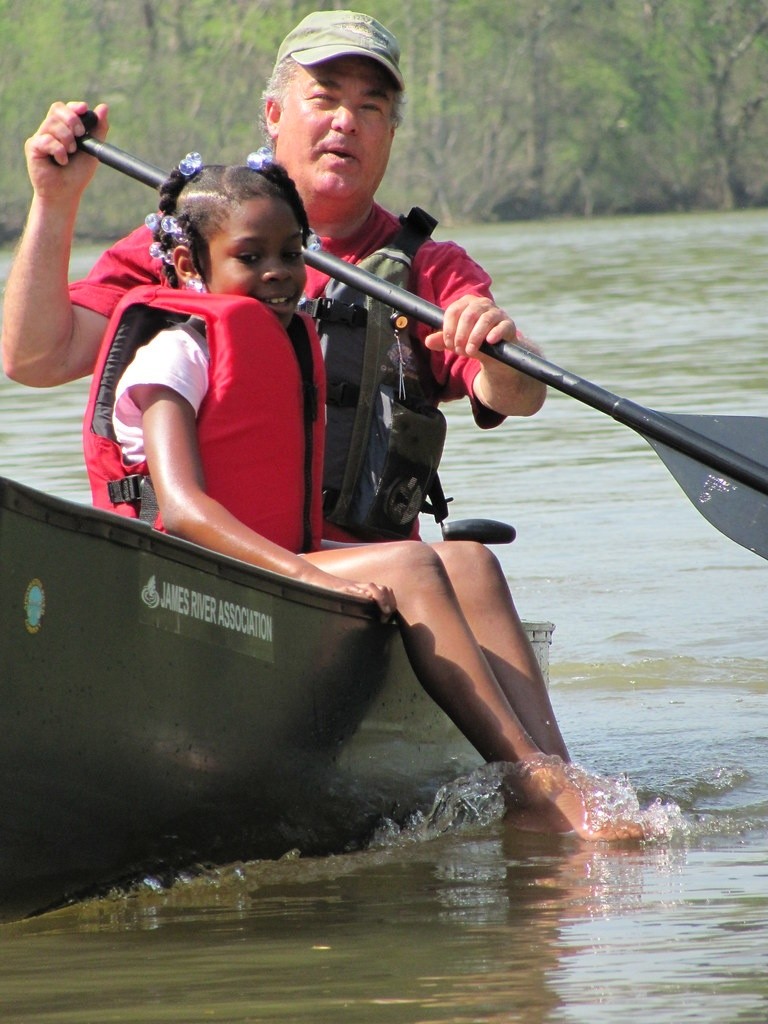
[272,11,405,92]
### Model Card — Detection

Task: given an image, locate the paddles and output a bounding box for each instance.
[43,110,768,556]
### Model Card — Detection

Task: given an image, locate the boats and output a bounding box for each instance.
[0,474,555,936]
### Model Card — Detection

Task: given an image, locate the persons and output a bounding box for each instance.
[2,9,547,543]
[85,146,650,843]
